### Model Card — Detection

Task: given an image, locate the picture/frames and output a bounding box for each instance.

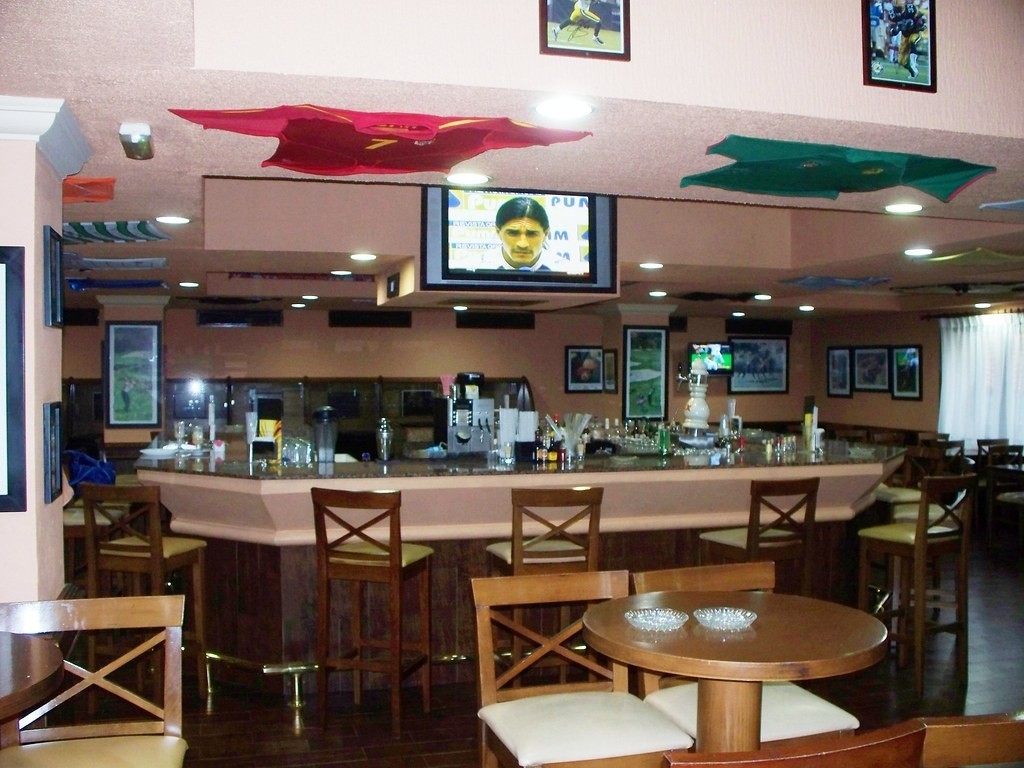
[44,224,64,327]
[862,0,937,93]
[622,324,668,422]
[538,0,630,61]
[891,344,923,401]
[727,336,789,395]
[852,345,891,392]
[827,346,852,399]
[105,319,161,427]
[603,349,617,393]
[565,345,604,392]
[44,401,62,504]
[0,246,27,512]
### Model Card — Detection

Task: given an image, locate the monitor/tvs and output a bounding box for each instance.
[687,341,734,377]
[419,186,618,294]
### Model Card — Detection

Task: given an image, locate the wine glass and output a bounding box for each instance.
[174,422,185,451]
[192,428,203,456]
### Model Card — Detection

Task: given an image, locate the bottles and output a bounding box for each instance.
[283,437,313,463]
[642,421,669,443]
[582,426,590,447]
[377,418,392,460]
[548,432,558,461]
[670,422,679,441]
[763,433,797,452]
[612,418,622,447]
[576,440,585,459]
[591,417,601,442]
[632,420,641,441]
[603,418,611,440]
[622,421,632,443]
[539,436,548,462]
[538,415,559,449]
[558,439,567,462]
[532,431,542,463]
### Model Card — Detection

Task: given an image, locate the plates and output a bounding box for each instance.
[140,449,178,456]
[625,608,689,631]
[694,607,757,630]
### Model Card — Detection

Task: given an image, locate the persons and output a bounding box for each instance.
[121,379,135,413]
[695,345,771,384]
[474,197,571,271]
[871,1,927,79]
[552,0,605,45]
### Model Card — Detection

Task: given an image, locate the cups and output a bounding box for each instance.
[496,441,515,471]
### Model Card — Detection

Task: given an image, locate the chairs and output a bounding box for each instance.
[0,427,1023,768]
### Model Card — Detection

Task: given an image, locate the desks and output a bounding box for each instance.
[0,632,63,721]
[583,589,888,754]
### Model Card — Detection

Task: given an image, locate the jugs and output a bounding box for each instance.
[313,404,338,460]
